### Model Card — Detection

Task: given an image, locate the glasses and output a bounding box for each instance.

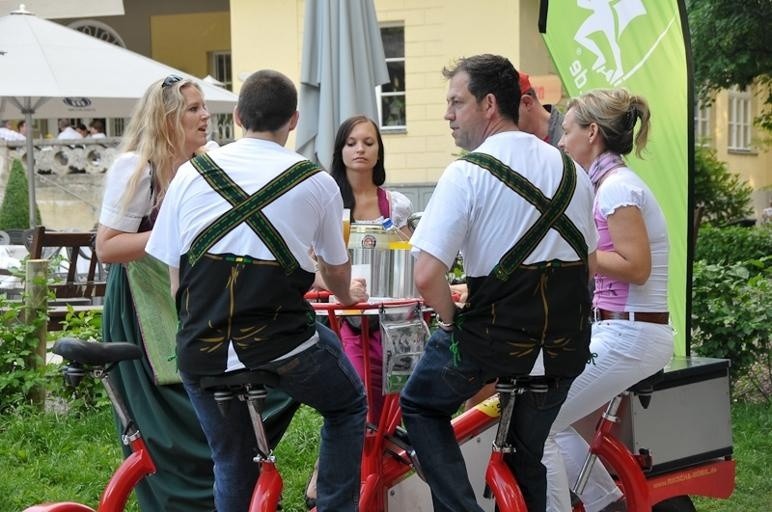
[161,72,183,90]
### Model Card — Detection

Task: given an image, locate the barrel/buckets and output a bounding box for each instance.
[370,249,422,298]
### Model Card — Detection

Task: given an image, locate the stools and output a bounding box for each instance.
[482,370,561,512]
[51,340,156,512]
[199,367,283,512]
[574,366,663,512]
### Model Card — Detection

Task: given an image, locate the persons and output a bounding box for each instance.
[146,69,366,512]
[89,120,106,138]
[399,54,597,512]
[517,70,568,154]
[95,77,302,512]
[75,124,91,139]
[17,120,27,137]
[55,117,84,148]
[0,119,26,140]
[305,118,416,510]
[540,88,676,512]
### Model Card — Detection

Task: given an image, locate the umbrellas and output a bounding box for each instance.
[0,3,240,230]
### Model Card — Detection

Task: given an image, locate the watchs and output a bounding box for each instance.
[436,313,455,328]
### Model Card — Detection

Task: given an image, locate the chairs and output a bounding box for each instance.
[12,227,112,333]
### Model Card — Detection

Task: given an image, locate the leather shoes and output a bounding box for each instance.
[305,472,316,510]
